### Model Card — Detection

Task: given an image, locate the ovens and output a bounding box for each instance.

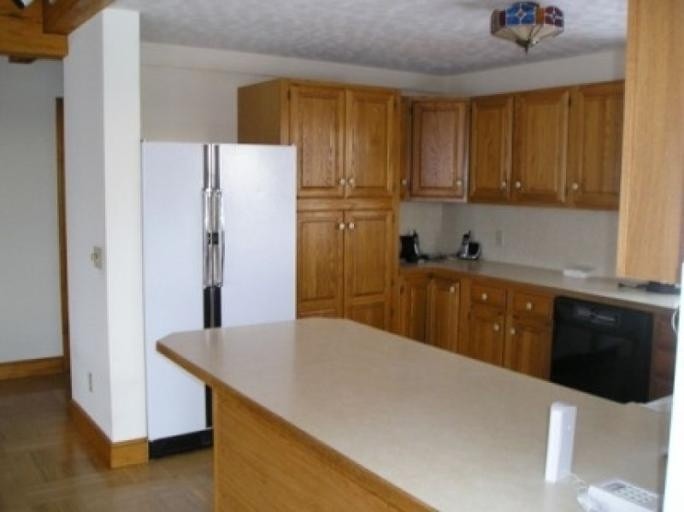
[550,296,653,405]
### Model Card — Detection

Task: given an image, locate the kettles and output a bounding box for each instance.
[456,232,482,259]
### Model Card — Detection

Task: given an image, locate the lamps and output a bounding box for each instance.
[488,2,565,56]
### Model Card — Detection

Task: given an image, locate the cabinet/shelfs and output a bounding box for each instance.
[400,273,430,344]
[567,80,625,211]
[428,274,461,354]
[466,85,568,209]
[647,312,676,402]
[398,94,468,202]
[616,2,682,285]
[462,277,554,384]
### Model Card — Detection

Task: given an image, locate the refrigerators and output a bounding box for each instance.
[140,140,298,460]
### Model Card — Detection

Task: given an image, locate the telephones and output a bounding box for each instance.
[457,230,480,260]
[405,230,428,263]
